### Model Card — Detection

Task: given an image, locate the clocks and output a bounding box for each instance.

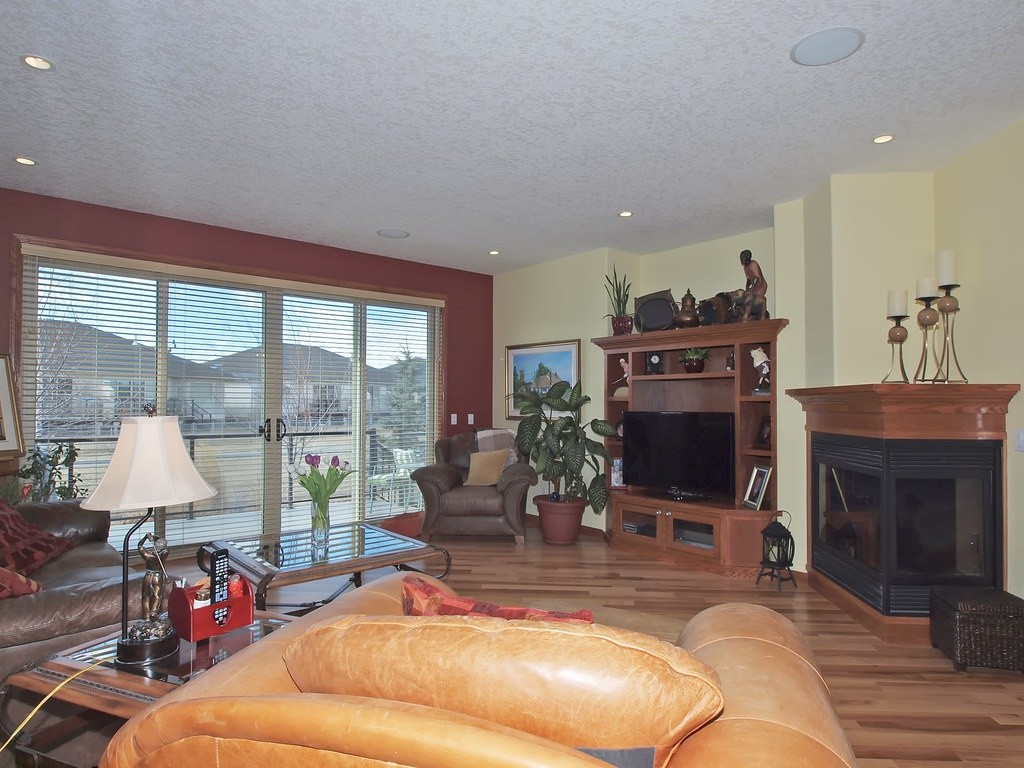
[645,352,664,374]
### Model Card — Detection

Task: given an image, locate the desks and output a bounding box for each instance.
[0,522,452,768]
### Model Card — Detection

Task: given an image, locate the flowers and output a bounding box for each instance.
[286,453,363,533]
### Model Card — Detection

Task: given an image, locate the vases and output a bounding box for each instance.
[310,502,330,549]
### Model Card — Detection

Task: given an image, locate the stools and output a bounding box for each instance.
[928,585,1024,672]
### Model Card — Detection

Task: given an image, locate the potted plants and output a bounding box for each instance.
[678,347,712,373]
[600,261,645,336]
[504,381,617,546]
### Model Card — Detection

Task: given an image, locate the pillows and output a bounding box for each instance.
[402,577,593,624]
[0,499,75,598]
[463,448,509,486]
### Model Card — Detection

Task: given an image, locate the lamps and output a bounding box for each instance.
[755,510,798,592]
[79,402,219,665]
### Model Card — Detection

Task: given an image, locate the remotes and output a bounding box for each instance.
[211,548,229,626]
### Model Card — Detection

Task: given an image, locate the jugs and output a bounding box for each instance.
[672,289,702,326]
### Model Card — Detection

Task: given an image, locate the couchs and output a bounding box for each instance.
[410,427,538,545]
[96,571,857,768]
[0,496,192,768]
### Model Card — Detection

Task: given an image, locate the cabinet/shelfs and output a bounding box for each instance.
[590,319,1021,646]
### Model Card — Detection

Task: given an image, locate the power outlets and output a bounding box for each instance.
[1015,432,1024,452]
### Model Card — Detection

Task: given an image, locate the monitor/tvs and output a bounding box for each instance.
[621,411,735,503]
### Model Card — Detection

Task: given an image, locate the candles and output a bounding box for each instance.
[887,259,957,315]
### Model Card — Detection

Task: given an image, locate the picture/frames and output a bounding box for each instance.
[505,339,581,423]
[608,456,633,495]
[742,463,773,511]
[0,353,27,457]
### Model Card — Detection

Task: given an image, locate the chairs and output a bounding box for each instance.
[366,448,427,517]
